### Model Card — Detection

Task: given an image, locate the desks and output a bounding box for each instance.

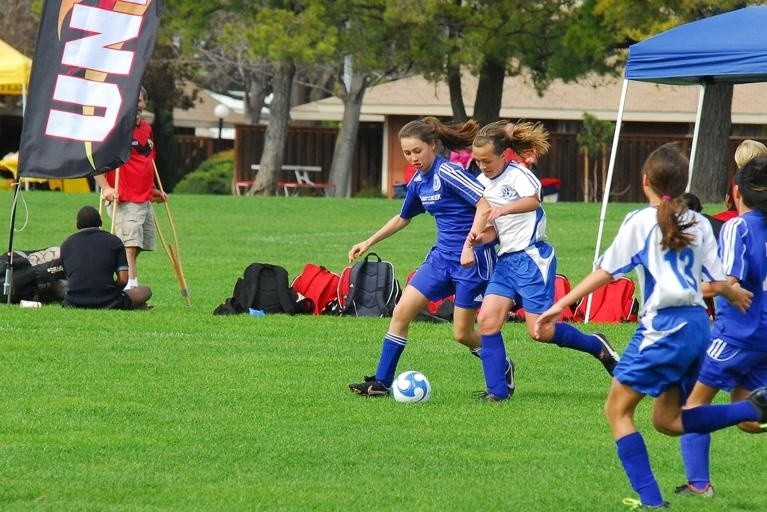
[250,164,322,197]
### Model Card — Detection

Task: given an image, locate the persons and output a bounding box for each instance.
[676,157,766,500]
[60,206,152,310]
[534,142,766,512]
[465,119,621,404]
[347,117,516,401]
[93,84,168,292]
[680,138,766,321]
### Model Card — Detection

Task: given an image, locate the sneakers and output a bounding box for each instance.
[348,375,391,397]
[504,355,514,396]
[674,480,715,498]
[622,497,670,511]
[743,385,766,423]
[471,390,510,403]
[590,331,620,377]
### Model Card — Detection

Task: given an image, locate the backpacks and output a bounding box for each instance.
[516,274,574,322]
[573,276,639,323]
[214,263,295,316]
[290,264,341,315]
[345,253,401,317]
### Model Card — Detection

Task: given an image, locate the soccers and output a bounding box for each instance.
[391,370,431,403]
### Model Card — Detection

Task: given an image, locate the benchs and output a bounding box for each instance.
[234,180,254,196]
[272,181,336,198]
